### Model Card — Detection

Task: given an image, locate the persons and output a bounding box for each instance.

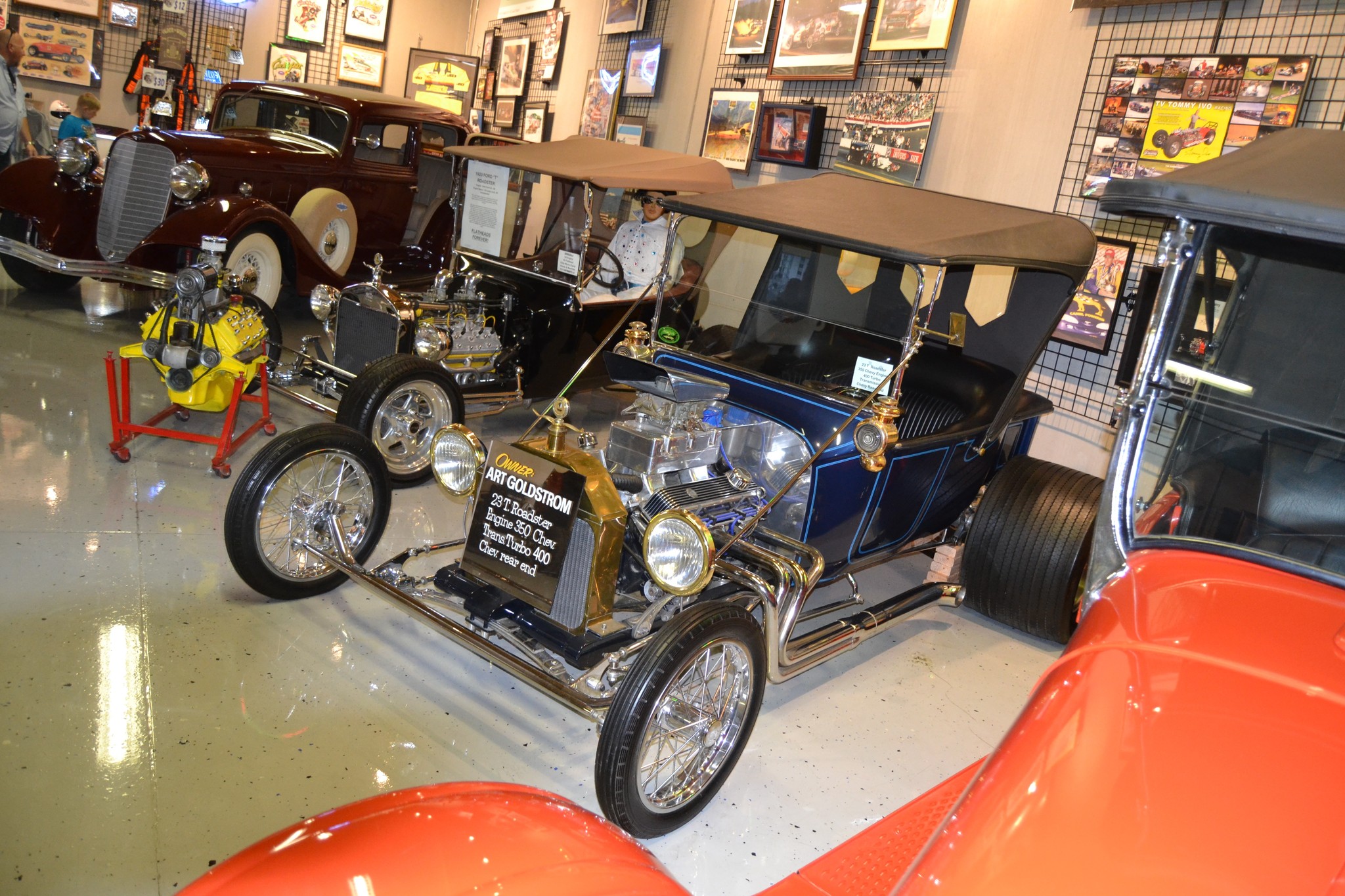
[1086,248,1123,299]
[0,27,39,175]
[574,185,686,302]
[56,93,105,170]
[1079,52,1318,202]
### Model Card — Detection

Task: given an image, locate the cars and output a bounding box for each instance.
[1,77,538,333]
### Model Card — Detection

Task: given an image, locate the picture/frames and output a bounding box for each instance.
[108,0,141,29]
[493,97,516,128]
[1051,236,1137,357]
[869,0,958,51]
[578,69,624,140]
[343,0,392,43]
[622,37,664,96]
[404,47,481,123]
[612,115,647,146]
[766,0,872,80]
[598,0,648,36]
[494,34,532,97]
[484,71,496,100]
[699,88,764,174]
[14,0,102,20]
[266,42,310,84]
[1116,264,1233,405]
[481,29,495,68]
[476,78,486,99]
[337,42,386,88]
[725,0,776,54]
[522,100,549,143]
[1079,55,1318,199]
[468,107,484,134]
[755,103,817,166]
[285,0,332,48]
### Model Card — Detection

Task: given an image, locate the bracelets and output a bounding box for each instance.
[24,140,35,148]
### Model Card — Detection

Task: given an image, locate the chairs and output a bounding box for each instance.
[1241,429,1344,576]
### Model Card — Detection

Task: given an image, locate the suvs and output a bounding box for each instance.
[219,163,1119,845]
[168,110,1343,894]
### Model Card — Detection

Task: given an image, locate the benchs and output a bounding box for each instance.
[354,141,456,245]
[762,317,1016,441]
[501,232,702,378]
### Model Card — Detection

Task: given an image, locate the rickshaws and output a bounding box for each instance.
[208,132,740,500]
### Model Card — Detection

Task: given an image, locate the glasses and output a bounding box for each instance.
[643,194,663,207]
[7,28,17,49]
